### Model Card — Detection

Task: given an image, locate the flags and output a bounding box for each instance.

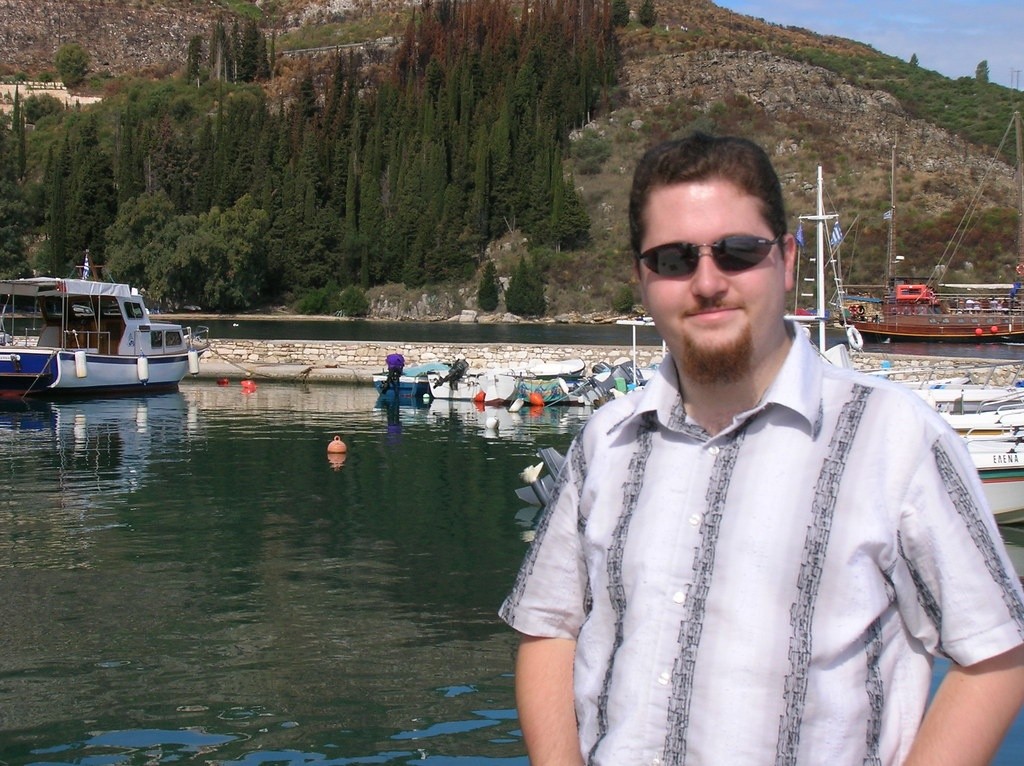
[830,221,841,246]
[796,222,804,248]
[82,257,89,280]
[883,210,892,219]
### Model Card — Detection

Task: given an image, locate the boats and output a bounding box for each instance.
[371,353,452,401]
[427,354,660,405]
[781,165,1024,524]
[0,276,211,393]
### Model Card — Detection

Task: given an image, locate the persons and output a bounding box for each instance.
[498,130,1024,766]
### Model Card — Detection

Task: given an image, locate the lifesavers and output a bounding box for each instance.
[847,327,863,350]
[1016,264,1024,274]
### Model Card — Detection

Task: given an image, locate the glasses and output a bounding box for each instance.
[639,232,782,276]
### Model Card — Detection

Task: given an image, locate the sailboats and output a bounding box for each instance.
[829,111,1024,341]
